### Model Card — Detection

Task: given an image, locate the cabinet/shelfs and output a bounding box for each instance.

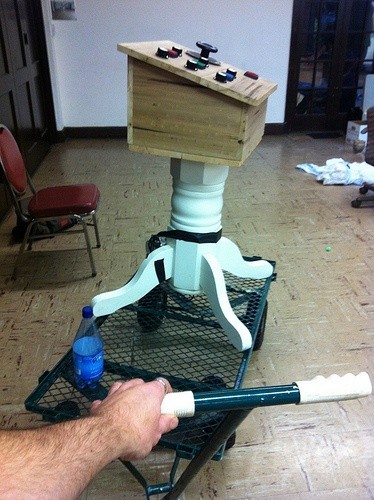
[285,0,374,128]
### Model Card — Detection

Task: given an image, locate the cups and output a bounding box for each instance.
[354,140,365,153]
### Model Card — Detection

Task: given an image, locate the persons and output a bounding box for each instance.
[0,377,179,500]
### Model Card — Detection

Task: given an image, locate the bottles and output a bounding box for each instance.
[72,305,104,390]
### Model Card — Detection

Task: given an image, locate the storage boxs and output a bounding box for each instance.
[345,121,368,147]
[300,62,325,85]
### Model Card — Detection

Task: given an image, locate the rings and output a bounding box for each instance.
[155,378,166,388]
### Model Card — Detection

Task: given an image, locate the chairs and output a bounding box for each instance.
[0,124,101,278]
